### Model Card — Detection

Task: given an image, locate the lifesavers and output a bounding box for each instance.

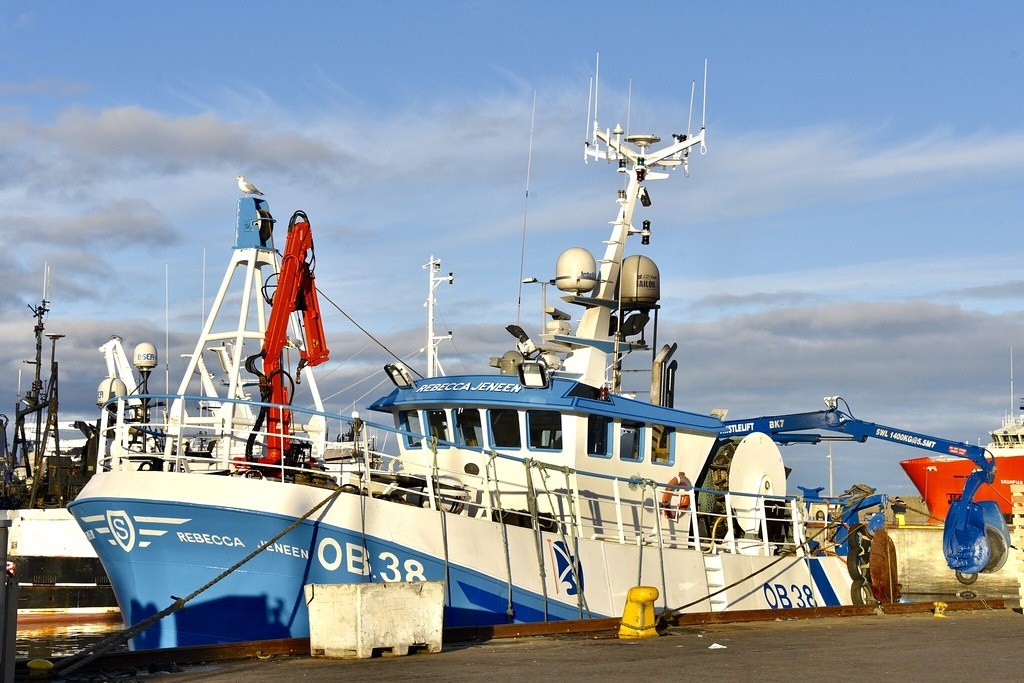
[661,475,692,521]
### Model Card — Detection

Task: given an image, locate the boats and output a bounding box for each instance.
[899,392,1024,522]
[0,260,145,643]
[60,33,1012,631]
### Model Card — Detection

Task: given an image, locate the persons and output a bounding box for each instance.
[890,496,907,514]
